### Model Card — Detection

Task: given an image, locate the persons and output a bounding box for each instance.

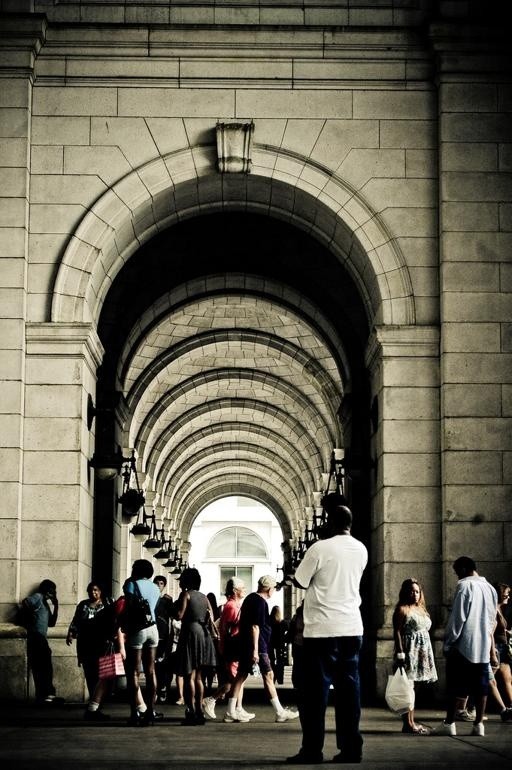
[66,582,113,718]
[11,579,65,705]
[286,505,366,764]
[114,559,308,726]
[393,578,439,737]
[434,556,512,737]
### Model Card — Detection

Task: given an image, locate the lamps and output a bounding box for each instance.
[162,536,176,567]
[300,526,312,558]
[170,547,183,576]
[153,524,168,559]
[321,452,352,505]
[306,510,320,548]
[121,450,145,516]
[176,562,189,581]
[132,492,151,537]
[285,547,298,580]
[145,509,160,549]
[313,508,329,535]
[292,538,308,571]
[94,452,118,482]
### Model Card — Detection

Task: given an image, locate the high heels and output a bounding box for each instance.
[144,708,157,727]
[185,706,195,723]
[195,706,206,726]
[128,710,140,727]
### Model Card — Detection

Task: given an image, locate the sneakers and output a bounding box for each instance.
[402,723,430,734]
[201,696,217,720]
[176,698,185,705]
[285,748,325,764]
[330,749,363,763]
[236,707,256,720]
[465,708,489,721]
[431,722,456,736]
[160,685,168,703]
[455,710,475,723]
[84,711,111,721]
[224,711,251,723]
[470,722,485,737]
[500,707,512,721]
[275,709,300,723]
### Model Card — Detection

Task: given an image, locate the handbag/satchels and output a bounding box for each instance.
[118,597,155,634]
[207,617,220,641]
[97,653,125,679]
[168,617,182,644]
